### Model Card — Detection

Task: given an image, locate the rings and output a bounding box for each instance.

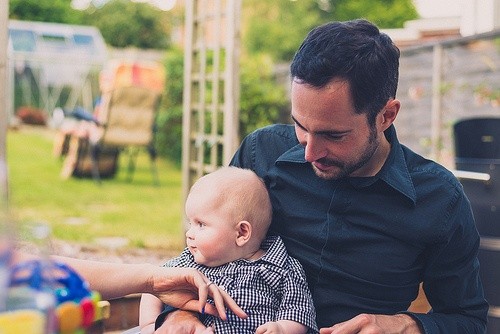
[206,281,215,287]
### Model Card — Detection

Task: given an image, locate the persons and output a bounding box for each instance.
[138,165,319,334]
[227,18,490,334]
[0,247,249,320]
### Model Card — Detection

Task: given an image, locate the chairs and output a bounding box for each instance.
[57,61,166,186]
